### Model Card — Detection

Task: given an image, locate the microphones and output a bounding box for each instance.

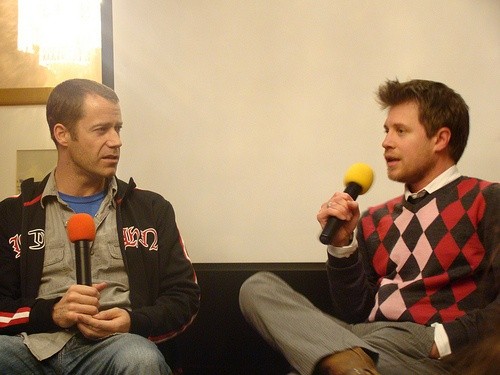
[319,164,373,244]
[67,213,96,286]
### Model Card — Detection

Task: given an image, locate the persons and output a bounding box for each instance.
[238,76,500,375]
[0,79,201,375]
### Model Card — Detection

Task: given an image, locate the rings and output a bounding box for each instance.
[327,202,331,208]
